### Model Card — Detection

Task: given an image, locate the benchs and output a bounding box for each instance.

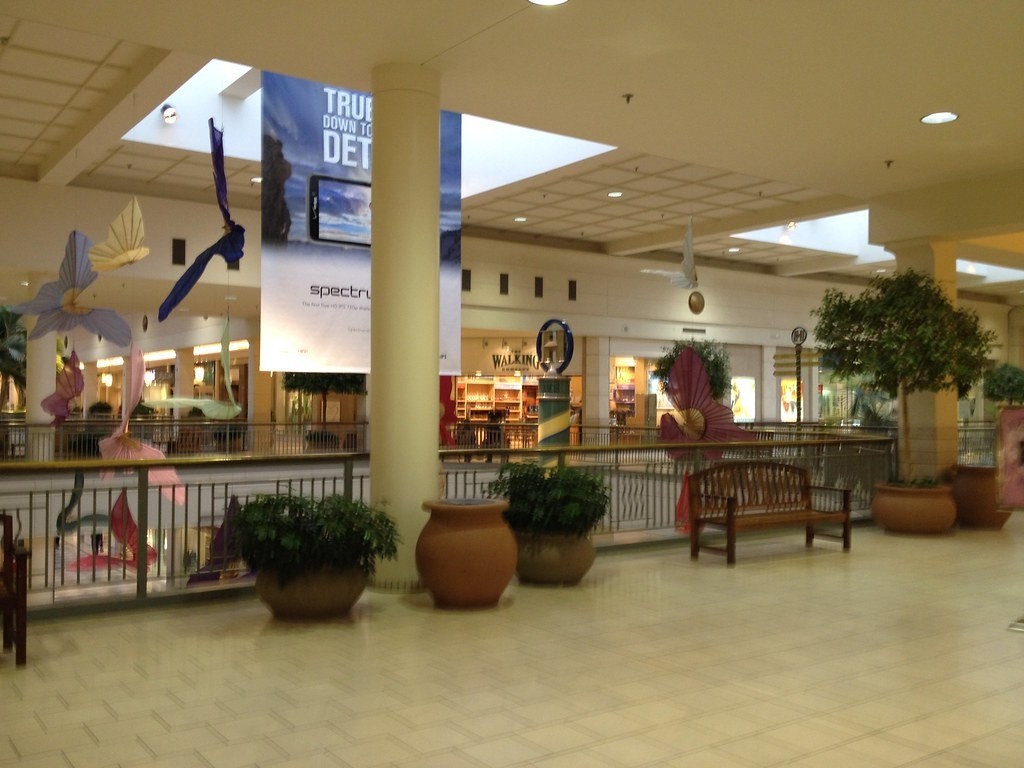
[688,457,852,565]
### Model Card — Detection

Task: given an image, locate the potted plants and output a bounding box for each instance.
[488,458,609,587]
[225,491,401,619]
[816,268,1000,533]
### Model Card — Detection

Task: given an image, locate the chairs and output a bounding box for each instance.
[0,514,28,666]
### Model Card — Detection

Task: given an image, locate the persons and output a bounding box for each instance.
[55,537,59,548]
[184,550,196,569]
[92,535,103,555]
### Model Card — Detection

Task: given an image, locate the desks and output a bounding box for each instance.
[946,461,1013,529]
[418,500,517,609]
